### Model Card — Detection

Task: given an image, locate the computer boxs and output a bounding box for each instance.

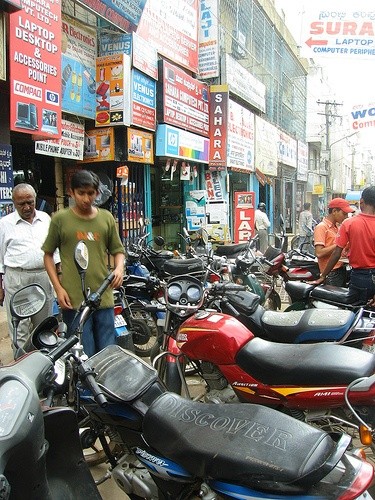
[29,103,36,129]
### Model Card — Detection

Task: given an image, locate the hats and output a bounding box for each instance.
[328,198,355,213]
[258,203,266,207]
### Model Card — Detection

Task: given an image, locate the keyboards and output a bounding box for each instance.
[14,122,28,129]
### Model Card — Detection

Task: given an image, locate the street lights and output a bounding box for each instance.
[327,131,359,204]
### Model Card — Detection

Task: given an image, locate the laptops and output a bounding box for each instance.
[41,109,59,134]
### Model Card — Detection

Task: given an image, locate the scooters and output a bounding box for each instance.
[11,240,373,500]
[0,336,103,500]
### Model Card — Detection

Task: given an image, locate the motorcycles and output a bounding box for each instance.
[115,231,375,445]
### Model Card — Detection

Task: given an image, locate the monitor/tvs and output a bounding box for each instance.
[145,138,152,150]
[101,135,110,146]
[14,102,30,122]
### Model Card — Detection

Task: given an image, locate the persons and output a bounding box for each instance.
[298,202,315,255]
[254,202,271,254]
[313,198,355,288]
[306,186,375,317]
[0,183,63,355]
[41,170,126,358]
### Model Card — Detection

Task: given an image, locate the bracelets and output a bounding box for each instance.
[320,273,328,279]
[57,271,62,276]
